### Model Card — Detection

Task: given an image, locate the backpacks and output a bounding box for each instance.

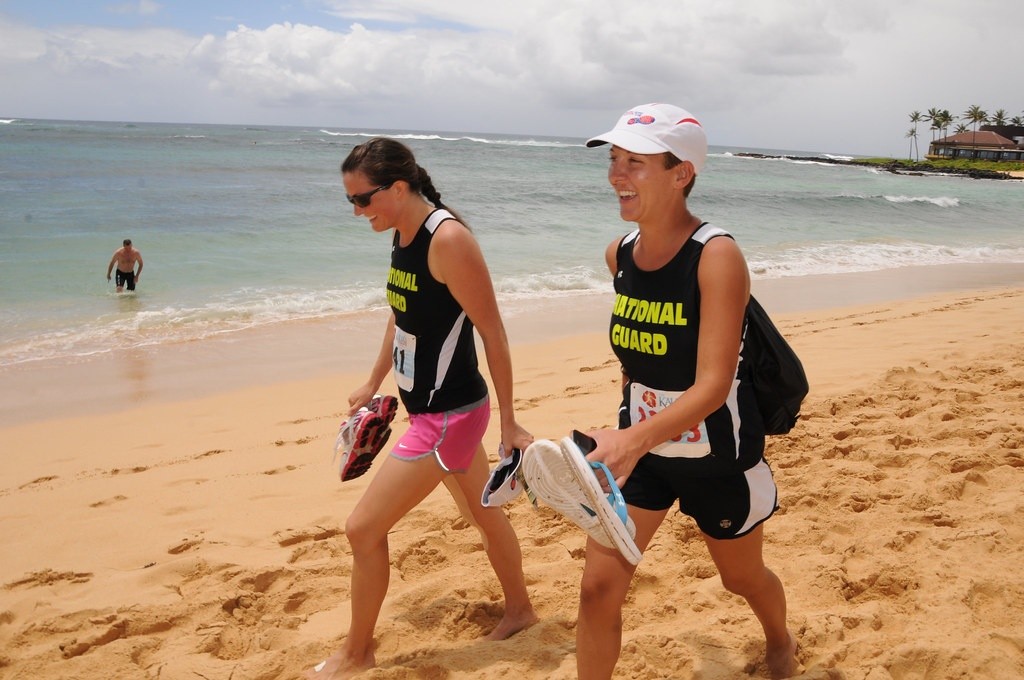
[748,296,809,436]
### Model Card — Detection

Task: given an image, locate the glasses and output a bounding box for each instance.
[345,186,392,208]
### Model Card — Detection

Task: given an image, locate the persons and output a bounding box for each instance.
[107,239,143,293]
[303,137,535,680]
[576,105,796,680]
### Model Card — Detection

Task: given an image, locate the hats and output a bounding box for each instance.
[584,103,707,176]
[480,444,524,505]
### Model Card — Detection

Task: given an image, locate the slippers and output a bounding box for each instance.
[524,437,636,549]
[561,437,644,567]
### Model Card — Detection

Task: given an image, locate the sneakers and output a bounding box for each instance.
[368,396,398,449]
[336,407,383,482]
